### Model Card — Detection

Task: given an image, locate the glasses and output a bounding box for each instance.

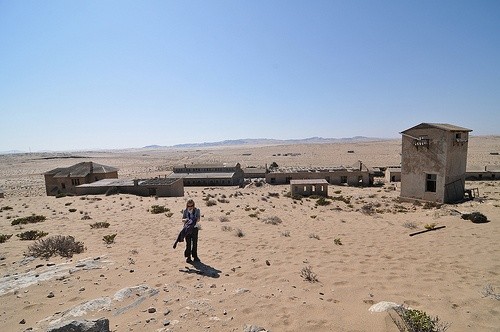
[188,205,194,207]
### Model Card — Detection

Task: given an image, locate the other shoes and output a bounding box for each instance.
[187,258,191,263]
[194,258,200,262]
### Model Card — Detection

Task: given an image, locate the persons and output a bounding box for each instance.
[182,199,201,263]
